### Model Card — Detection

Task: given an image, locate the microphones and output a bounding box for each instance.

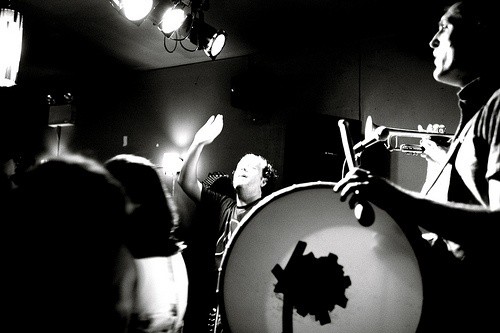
[352,126,389,154]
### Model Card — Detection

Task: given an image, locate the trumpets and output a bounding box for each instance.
[364,115,454,157]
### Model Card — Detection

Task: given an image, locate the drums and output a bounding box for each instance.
[215,181,424,333]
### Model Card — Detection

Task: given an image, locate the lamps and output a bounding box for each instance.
[110,0,228,62]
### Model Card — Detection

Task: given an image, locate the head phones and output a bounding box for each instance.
[263,166,279,178]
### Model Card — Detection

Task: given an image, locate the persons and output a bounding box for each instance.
[1,148,187,333]
[417,124,451,243]
[178,113,279,333]
[334,0,500,333]
